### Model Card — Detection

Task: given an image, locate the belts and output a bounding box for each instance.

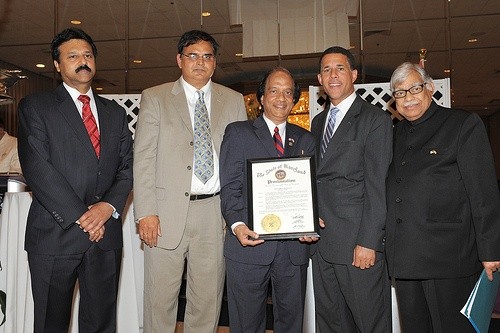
[190,189,220,202]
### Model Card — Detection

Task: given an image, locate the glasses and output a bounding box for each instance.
[392,80,428,99]
[180,51,216,60]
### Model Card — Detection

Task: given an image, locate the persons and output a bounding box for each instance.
[0,125,26,183]
[219,67,325,333]
[311,46,394,333]
[17,27,133,333]
[133,29,249,333]
[385,62,500,333]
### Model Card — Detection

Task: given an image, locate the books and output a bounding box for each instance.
[459,268,500,333]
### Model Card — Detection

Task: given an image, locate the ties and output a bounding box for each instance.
[320,107,340,163]
[273,126,284,157]
[76,94,101,161]
